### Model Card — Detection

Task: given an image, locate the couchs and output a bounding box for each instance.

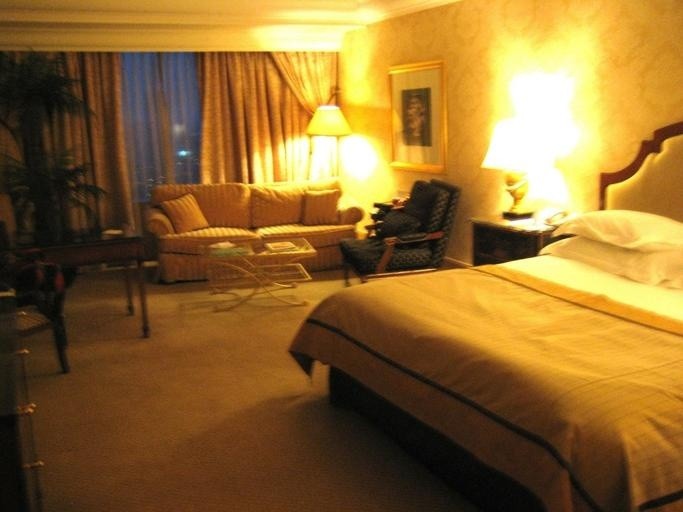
[139,179,363,282]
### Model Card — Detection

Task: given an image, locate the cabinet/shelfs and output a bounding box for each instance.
[0,221,47,511]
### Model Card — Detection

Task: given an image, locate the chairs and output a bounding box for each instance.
[340,178,460,290]
[1,257,71,374]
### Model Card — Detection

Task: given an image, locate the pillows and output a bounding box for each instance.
[550,208,683,252]
[380,210,422,247]
[159,192,209,234]
[538,235,683,289]
[301,188,341,226]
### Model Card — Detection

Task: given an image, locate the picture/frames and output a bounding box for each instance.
[386,59,449,176]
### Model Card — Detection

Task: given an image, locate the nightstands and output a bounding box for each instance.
[470,215,576,264]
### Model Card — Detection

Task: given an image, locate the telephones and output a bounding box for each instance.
[544,211,573,227]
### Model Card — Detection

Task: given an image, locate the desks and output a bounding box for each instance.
[197,233,319,312]
[0,221,155,342]
[369,198,400,225]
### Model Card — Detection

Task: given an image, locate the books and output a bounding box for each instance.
[263,241,298,252]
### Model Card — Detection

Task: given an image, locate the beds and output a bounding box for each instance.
[287,118,683,512]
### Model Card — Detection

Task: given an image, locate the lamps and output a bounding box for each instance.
[307,103,352,175]
[479,116,545,218]
